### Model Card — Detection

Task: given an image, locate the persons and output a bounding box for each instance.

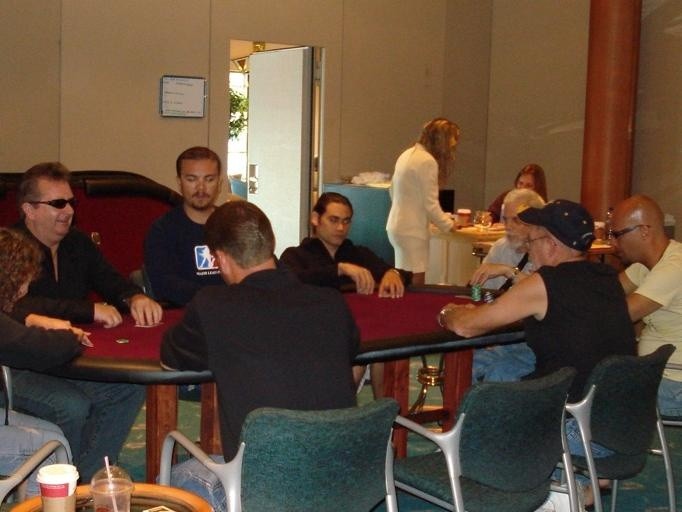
[610,193,682,486]
[385,118,463,287]
[486,164,550,225]
[437,199,638,508]
[469,188,551,388]
[6,161,165,484]
[286,192,414,391]
[0,223,94,500]
[142,146,280,310]
[156,201,368,512]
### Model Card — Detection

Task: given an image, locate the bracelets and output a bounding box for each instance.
[509,267,520,281]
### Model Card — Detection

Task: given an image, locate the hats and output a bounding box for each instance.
[517,197,595,253]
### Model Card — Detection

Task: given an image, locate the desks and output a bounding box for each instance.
[21,282,528,481]
[473,238,612,265]
[320,184,455,269]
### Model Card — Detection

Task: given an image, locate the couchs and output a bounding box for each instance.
[0,169,183,275]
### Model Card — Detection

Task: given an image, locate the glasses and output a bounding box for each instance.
[611,224,650,239]
[525,235,559,251]
[28,197,78,209]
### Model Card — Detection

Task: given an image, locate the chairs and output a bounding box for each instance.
[562,343,679,510]
[159,397,400,511]
[128,264,178,309]
[393,365,582,511]
[0,366,71,512]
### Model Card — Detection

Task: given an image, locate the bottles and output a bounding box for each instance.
[605,205,616,242]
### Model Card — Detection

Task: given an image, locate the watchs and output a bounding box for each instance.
[440,308,455,330]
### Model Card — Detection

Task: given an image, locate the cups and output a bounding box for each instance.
[456,208,472,227]
[91,472,135,512]
[36,464,80,512]
